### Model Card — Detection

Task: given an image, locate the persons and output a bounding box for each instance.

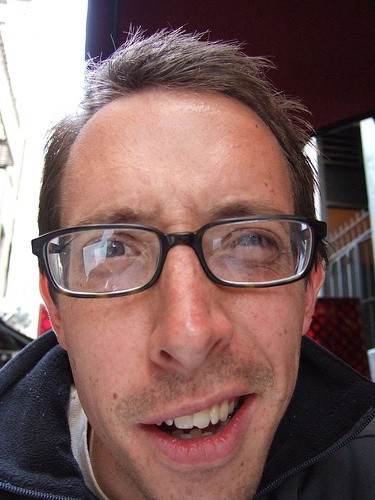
[0,28,373,500]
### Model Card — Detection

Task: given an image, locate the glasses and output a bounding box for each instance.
[31,213,327,298]
